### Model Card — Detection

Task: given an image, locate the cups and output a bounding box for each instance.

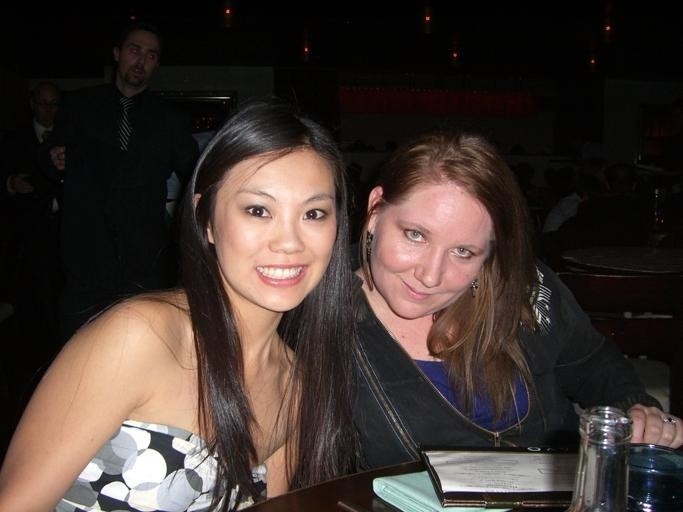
[617,444,682,510]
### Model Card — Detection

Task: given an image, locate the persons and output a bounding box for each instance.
[0,80,77,322]
[57,22,200,341]
[0,99,354,512]
[342,131,682,471]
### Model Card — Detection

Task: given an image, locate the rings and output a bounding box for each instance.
[663,416,677,426]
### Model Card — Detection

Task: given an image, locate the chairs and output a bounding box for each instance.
[556,272,681,423]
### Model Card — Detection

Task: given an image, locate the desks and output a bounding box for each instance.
[558,245,683,273]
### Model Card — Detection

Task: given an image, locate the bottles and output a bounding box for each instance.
[569,406,632,512]
